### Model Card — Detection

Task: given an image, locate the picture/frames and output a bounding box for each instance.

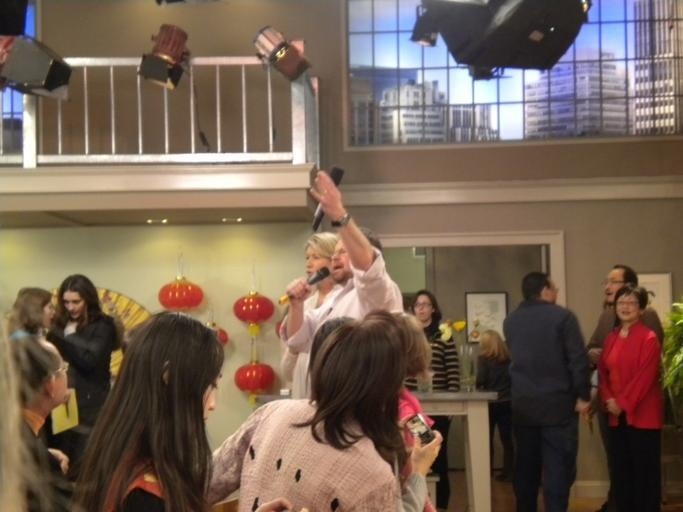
[634,271,675,335]
[464,290,509,344]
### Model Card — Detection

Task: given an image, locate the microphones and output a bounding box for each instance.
[279,267,329,306]
[311,165,345,232]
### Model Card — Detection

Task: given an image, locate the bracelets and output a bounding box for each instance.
[330,211,351,228]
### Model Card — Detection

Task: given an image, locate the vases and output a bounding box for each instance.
[455,341,480,393]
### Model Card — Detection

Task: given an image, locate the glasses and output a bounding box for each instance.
[601,279,623,285]
[55,362,69,376]
[617,300,639,306]
[415,303,432,307]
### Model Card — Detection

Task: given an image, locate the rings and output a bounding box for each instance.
[322,190,328,195]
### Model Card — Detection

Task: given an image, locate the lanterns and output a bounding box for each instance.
[204,323,227,347]
[233,290,276,335]
[231,358,275,402]
[158,276,202,314]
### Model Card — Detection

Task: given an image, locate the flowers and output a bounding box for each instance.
[438,316,480,384]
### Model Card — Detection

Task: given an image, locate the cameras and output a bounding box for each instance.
[406,413,435,444]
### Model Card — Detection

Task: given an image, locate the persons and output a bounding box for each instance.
[583,282,667,512]
[7,288,78,480]
[202,309,446,511]
[46,274,117,434]
[502,271,593,512]
[280,170,403,399]
[585,264,676,507]
[72,312,308,512]
[473,330,517,483]
[387,311,438,511]
[2,331,77,510]
[271,231,339,401]
[401,289,459,511]
[308,316,359,391]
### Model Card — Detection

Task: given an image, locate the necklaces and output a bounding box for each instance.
[621,327,631,335]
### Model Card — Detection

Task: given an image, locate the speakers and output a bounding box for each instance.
[422,0,592,80]
[0,32,72,100]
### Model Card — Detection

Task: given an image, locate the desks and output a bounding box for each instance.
[402,390,498,512]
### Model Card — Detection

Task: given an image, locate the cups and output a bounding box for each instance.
[460,344,480,391]
[418,376,433,395]
